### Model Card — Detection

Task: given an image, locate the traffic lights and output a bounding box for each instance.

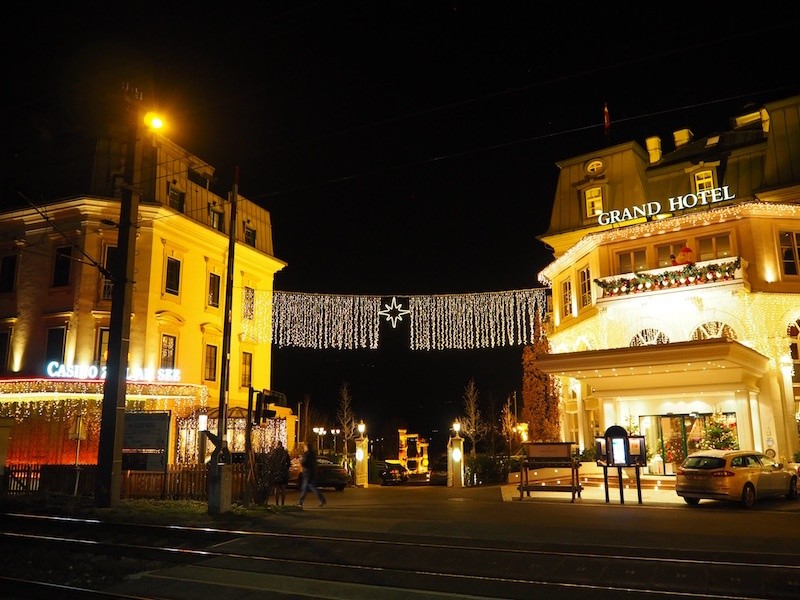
[255,393,277,425]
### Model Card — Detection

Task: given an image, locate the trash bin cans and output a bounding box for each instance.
[207,463,231,514]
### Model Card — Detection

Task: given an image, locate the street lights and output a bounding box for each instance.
[313,427,324,456]
[354,418,368,488]
[330,429,340,453]
[320,431,327,452]
[93,75,170,503]
[446,418,465,489]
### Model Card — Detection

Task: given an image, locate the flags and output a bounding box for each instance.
[601,103,610,135]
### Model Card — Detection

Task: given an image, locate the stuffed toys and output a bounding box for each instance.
[670,247,695,267]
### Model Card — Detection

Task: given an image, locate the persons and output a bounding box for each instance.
[271,441,290,506]
[295,444,327,509]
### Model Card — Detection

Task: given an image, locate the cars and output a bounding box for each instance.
[298,464,348,491]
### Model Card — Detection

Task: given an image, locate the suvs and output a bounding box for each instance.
[375,462,409,486]
[675,449,798,509]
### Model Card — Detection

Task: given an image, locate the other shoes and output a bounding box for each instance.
[293,503,302,507]
[319,499,327,506]
